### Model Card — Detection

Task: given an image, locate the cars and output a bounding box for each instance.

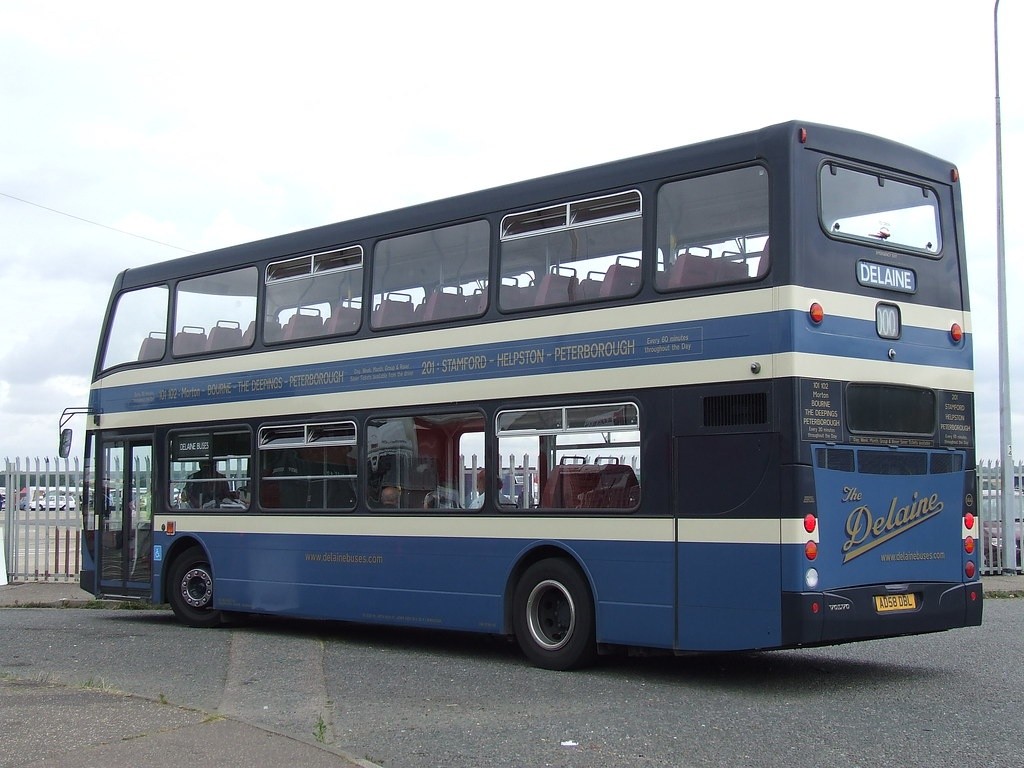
[981,488,1024,574]
[1,485,181,511]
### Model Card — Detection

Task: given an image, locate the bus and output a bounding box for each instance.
[58,119,984,671]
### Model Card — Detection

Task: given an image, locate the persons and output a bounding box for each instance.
[423,490,446,508]
[467,468,515,509]
[181,460,233,509]
[375,487,399,508]
